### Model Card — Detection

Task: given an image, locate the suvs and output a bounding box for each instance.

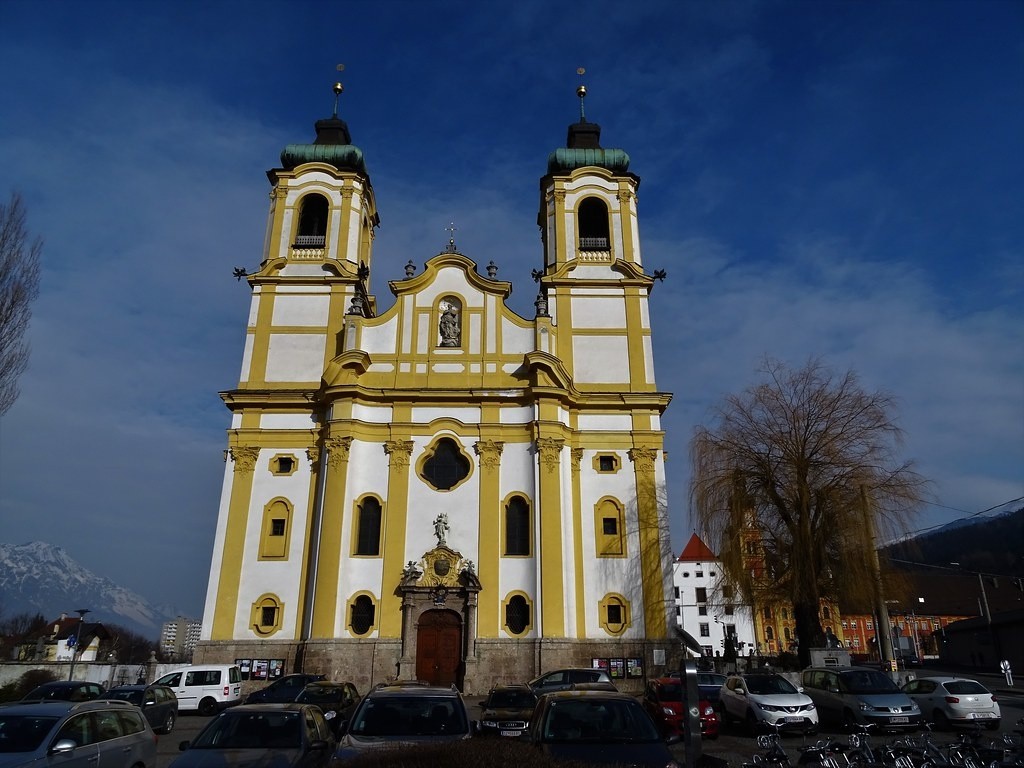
[479,681,538,744]
[717,670,819,737]
[0,699,159,768]
[527,668,619,695]
[801,666,922,734]
[329,683,479,768]
[662,673,729,702]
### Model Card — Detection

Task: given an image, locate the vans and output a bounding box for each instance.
[146,664,242,716]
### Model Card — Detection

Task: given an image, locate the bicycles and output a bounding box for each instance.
[740,722,1024,768]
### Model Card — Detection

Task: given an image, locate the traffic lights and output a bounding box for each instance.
[69,607,93,680]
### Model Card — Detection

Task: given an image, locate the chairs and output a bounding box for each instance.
[211,674,219,684]
[430,705,450,732]
[185,676,190,685]
[72,689,82,700]
[554,711,581,737]
[379,707,402,732]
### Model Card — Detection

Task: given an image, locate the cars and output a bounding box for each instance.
[164,702,336,768]
[645,679,719,740]
[901,677,1002,731]
[292,681,363,740]
[20,681,106,705]
[529,691,680,768]
[246,674,329,703]
[92,683,178,736]
